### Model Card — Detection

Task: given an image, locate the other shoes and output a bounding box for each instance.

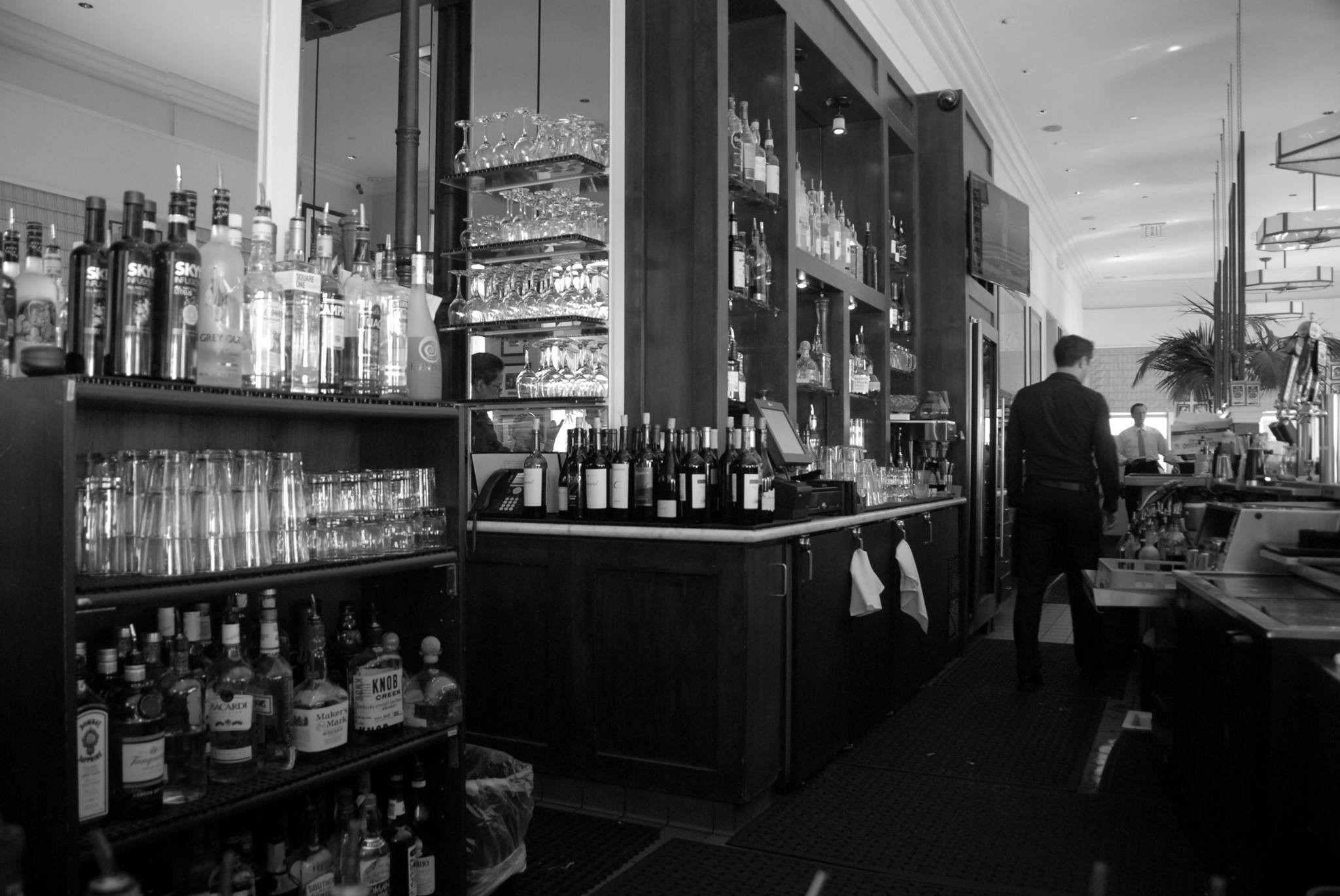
[1018,670,1042,690]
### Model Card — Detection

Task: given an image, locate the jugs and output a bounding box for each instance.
[919,390,951,419]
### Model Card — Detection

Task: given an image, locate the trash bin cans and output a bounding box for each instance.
[460,744,534,896]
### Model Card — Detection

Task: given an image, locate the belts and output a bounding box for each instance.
[1038,480,1088,490]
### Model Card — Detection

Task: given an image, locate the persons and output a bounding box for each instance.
[470,352,512,488]
[1003,335,1120,692]
[1115,403,1184,536]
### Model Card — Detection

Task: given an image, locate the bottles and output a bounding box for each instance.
[888,210,907,267]
[558,414,775,526]
[1195,435,1209,474]
[523,429,547,519]
[889,278,911,332]
[72,588,462,896]
[796,332,831,391]
[796,154,878,290]
[0,164,443,402]
[729,98,782,306]
[1117,509,1187,570]
[801,430,818,473]
[727,329,746,403]
[849,325,881,398]
[809,415,820,450]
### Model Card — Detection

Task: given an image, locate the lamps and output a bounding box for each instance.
[1245,251,1335,294]
[1275,109,1340,178]
[1244,256,1307,320]
[825,92,852,136]
[794,47,809,92]
[1256,171,1340,252]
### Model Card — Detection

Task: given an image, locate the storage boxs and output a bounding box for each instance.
[1231,422,1261,435]
[1169,432,1220,455]
[1098,554,1182,588]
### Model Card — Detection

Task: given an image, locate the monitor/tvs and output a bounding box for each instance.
[966,170,1031,295]
[746,398,812,468]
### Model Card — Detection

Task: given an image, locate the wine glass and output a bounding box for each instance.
[446,186,608,398]
[453,107,609,173]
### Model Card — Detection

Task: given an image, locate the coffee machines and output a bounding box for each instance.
[892,419,958,497]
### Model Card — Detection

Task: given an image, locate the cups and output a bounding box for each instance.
[849,417,864,447]
[74,451,448,578]
[914,470,930,499]
[1215,455,1234,479]
[819,444,914,507]
[890,341,917,373]
[1245,448,1267,488]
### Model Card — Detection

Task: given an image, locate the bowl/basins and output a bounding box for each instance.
[889,395,917,412]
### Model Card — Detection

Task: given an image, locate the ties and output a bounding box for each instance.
[1138,430,1145,458]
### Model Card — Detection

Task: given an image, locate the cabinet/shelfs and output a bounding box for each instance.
[0,374,471,896]
[440,152,613,415]
[627,0,924,486]
[896,506,970,714]
[1158,595,1340,896]
[782,518,895,800]
